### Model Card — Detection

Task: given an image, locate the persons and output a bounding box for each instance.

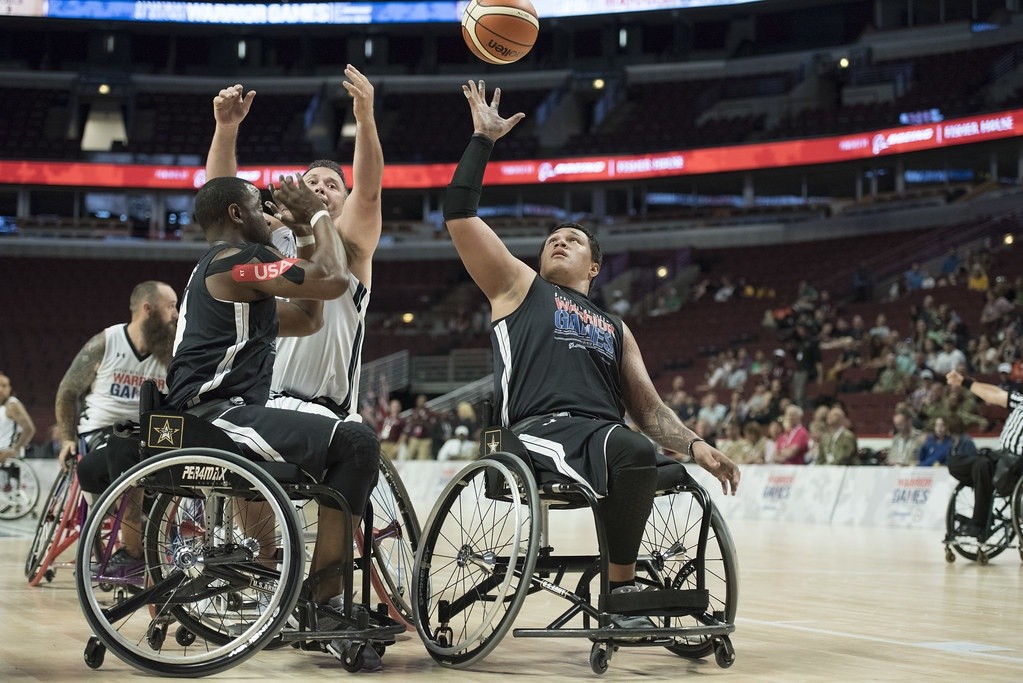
[165,64,385,631]
[357,249,1023,467]
[946,368,1023,541]
[58,280,179,578]
[444,79,740,641]
[0,374,34,463]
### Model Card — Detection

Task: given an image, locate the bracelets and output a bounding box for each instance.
[959,375,976,389]
[310,210,332,229]
[688,438,705,464]
[293,234,316,246]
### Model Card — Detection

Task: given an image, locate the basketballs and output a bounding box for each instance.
[460,0,540,65]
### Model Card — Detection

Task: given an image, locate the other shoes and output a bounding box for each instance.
[606,586,655,643]
[73,547,147,580]
[287,581,383,671]
[956,524,989,543]
[253,578,274,614]
[253,580,280,618]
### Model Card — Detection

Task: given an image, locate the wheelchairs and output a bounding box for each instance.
[410,422,740,676]
[0,454,41,522]
[943,468,1023,566]
[25,379,431,683]
